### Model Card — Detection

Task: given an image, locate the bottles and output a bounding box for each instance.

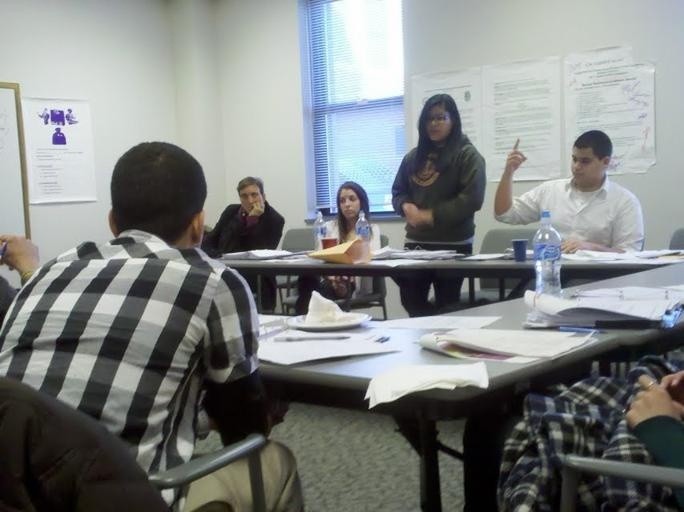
[355,210,372,261]
[312,210,327,251]
[533,212,565,307]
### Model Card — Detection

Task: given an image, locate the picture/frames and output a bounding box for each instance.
[0,82,30,290]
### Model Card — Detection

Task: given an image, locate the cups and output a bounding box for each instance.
[511,239,528,262]
[321,237,338,251]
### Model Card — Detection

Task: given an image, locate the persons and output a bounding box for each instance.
[1,141,304,512]
[461,368,684,512]
[1,233,41,330]
[201,176,286,312]
[294,180,381,317]
[492,129,647,302]
[390,93,487,316]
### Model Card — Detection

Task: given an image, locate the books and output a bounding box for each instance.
[221,248,294,260]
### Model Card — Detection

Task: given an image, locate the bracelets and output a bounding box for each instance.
[20,270,33,280]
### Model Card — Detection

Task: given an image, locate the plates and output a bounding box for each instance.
[285,310,370,331]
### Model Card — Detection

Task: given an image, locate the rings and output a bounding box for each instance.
[644,379,657,389]
